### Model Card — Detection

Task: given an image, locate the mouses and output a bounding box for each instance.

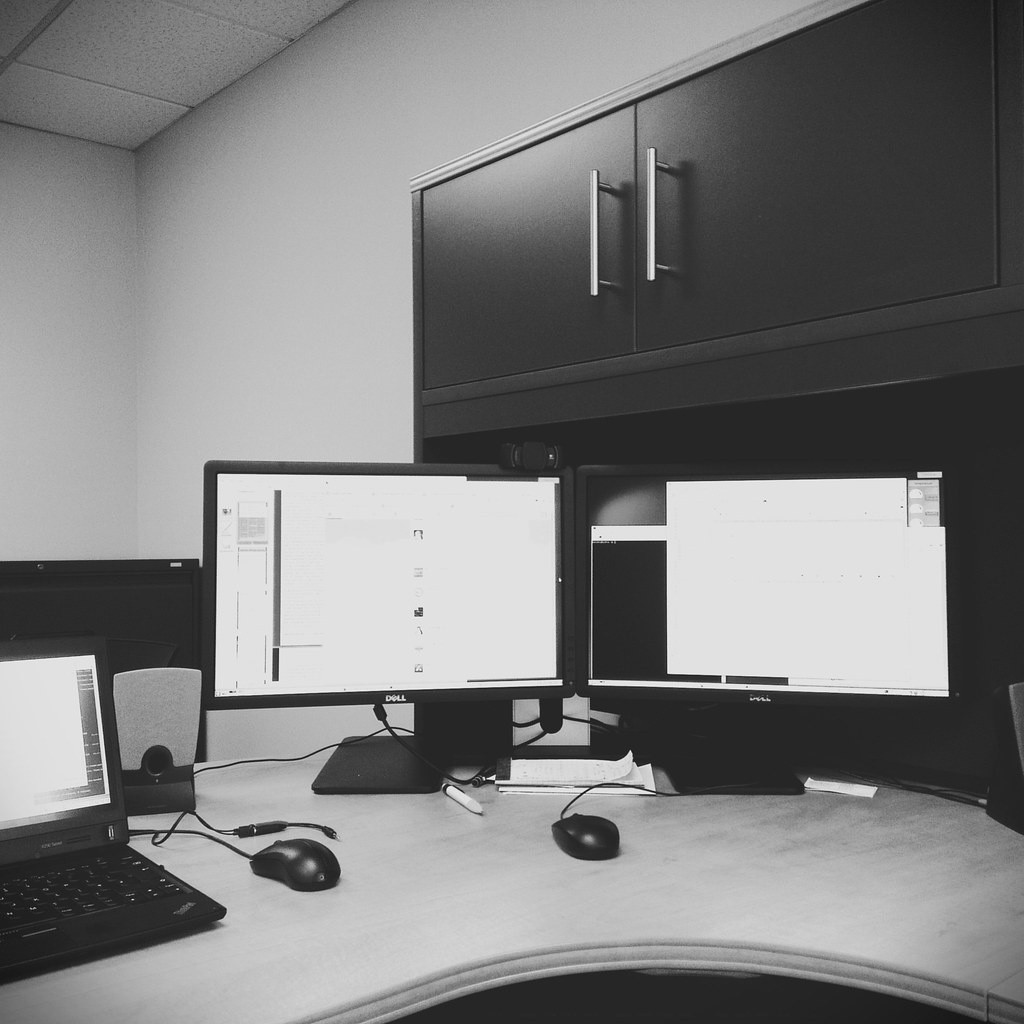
[250,838,342,893]
[551,813,619,861]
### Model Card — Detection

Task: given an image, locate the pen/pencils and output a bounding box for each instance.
[472,765,498,788]
[441,783,485,815]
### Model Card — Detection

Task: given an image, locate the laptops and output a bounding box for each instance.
[0,637,227,977]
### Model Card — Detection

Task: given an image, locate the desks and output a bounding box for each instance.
[0,752,1024,1024]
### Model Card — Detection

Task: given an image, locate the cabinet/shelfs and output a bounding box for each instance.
[414,0,1024,836]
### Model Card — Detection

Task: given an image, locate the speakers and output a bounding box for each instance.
[110,668,202,816]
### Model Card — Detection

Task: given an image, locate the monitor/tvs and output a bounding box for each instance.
[202,461,576,798]
[0,558,209,766]
[575,465,966,796]
